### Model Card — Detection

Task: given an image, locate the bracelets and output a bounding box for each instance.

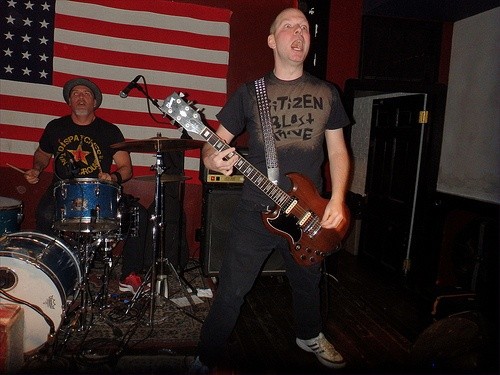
[113,172,122,184]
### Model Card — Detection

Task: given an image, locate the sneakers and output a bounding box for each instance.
[119,272,151,296]
[189,356,209,375]
[296,332,345,368]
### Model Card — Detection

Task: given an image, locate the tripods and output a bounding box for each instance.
[56,145,199,354]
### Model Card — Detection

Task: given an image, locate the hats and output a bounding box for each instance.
[62,79,102,108]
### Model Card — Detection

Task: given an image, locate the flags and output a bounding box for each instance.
[0,0,230,184]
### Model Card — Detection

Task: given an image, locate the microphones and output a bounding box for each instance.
[119,75,141,98]
[64,150,81,174]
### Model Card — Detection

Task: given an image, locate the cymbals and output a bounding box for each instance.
[110,137,205,155]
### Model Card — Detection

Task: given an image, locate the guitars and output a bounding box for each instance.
[158,90,352,268]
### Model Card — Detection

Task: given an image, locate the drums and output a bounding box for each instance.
[0,196,25,238]
[0,231,83,360]
[50,177,124,233]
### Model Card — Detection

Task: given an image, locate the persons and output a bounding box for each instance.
[193,7,351,375]
[25,78,150,297]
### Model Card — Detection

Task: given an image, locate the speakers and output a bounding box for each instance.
[199,186,288,276]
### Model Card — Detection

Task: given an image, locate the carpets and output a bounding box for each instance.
[45,260,239,353]
